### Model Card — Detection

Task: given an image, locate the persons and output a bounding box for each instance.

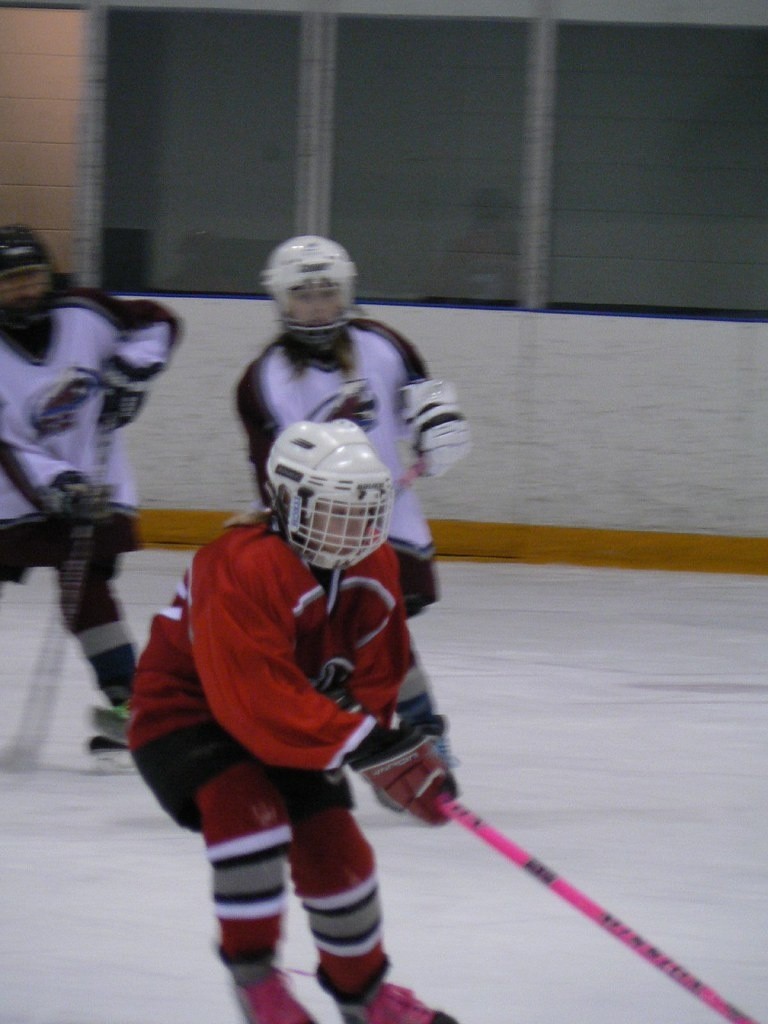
[126,419,458,1024]
[237,236,472,813]
[0,224,180,750]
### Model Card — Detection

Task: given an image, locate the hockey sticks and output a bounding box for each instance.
[83,459,426,771]
[435,795,760,1024]
[0,415,115,777]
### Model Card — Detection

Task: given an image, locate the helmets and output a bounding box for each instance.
[0,225,56,316]
[264,417,394,566]
[261,234,357,350]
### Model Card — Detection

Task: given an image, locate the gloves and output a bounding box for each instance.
[400,377,472,478]
[344,707,459,826]
[46,471,116,520]
[98,357,164,431]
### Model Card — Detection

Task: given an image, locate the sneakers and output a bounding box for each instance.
[87,705,137,774]
[220,940,314,1024]
[314,952,457,1024]
